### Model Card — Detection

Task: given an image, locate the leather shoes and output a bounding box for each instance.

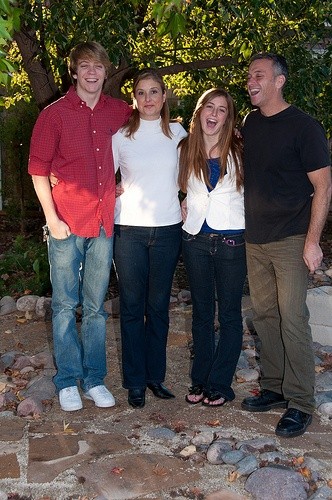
[240,388,288,412]
[276,407,313,437]
[146,382,175,399]
[128,388,146,408]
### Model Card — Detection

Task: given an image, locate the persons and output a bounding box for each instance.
[27,44,137,411]
[182,54,332,437]
[117,87,248,406]
[49,69,187,407]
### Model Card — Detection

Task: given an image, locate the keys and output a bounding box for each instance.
[43,229,49,242]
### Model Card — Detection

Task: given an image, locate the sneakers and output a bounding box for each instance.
[58,385,83,411]
[82,384,115,407]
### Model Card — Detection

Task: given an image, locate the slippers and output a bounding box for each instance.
[185,386,204,404]
[201,390,229,407]
